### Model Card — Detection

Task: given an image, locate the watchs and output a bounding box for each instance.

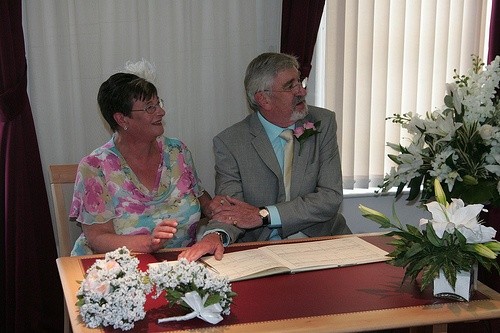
[258,206,270,226]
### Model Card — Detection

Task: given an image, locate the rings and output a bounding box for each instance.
[233,219,237,226]
[228,215,232,220]
[220,200,224,204]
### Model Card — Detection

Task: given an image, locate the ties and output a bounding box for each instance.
[279,129,294,203]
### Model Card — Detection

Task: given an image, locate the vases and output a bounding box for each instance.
[482,208,500,227]
[433,265,479,302]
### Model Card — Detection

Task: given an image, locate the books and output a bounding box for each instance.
[195,236,395,283]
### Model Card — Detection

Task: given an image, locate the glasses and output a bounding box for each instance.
[126,99,164,114]
[255,77,307,95]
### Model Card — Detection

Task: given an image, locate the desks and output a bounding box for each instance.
[56,231,500,333]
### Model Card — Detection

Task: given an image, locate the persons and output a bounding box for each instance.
[69,73,212,254]
[178,52,353,263]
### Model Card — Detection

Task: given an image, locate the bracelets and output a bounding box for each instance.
[215,231,227,243]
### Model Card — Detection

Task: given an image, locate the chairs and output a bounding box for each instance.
[49,165,79,333]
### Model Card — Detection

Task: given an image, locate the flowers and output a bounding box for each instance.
[75,246,238,331]
[293,120,323,157]
[359,52,500,302]
